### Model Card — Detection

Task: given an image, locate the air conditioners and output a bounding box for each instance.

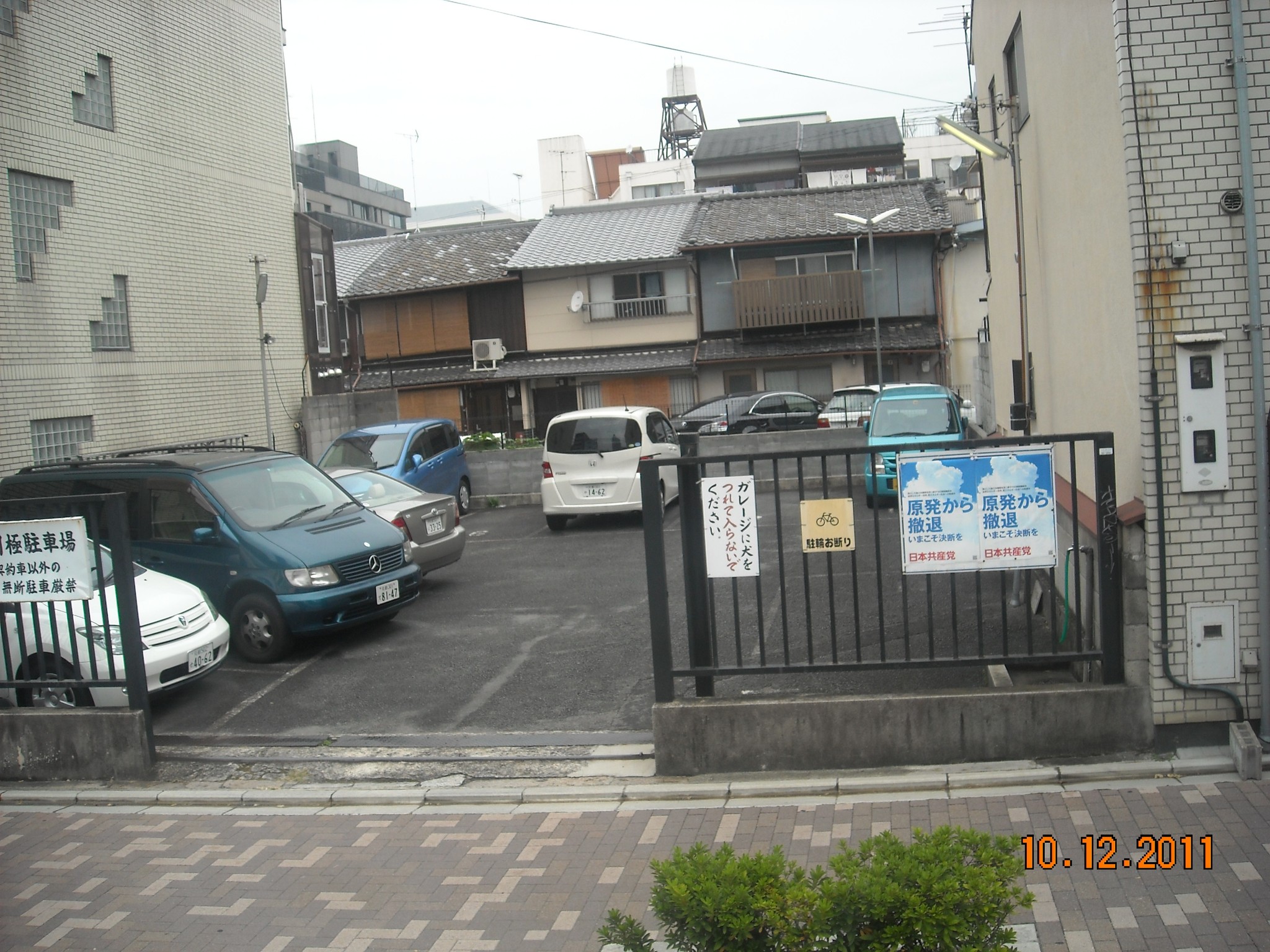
[472,338,505,360]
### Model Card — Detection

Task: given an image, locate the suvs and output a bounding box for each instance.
[817,381,977,430]
[0,441,423,664]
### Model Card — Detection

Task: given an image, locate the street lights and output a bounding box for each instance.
[834,207,902,389]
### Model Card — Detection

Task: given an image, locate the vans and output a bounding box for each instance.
[862,386,969,510]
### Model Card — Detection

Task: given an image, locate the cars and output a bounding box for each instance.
[668,389,827,437]
[317,416,472,516]
[269,465,466,578]
[540,406,681,533]
[0,538,231,708]
